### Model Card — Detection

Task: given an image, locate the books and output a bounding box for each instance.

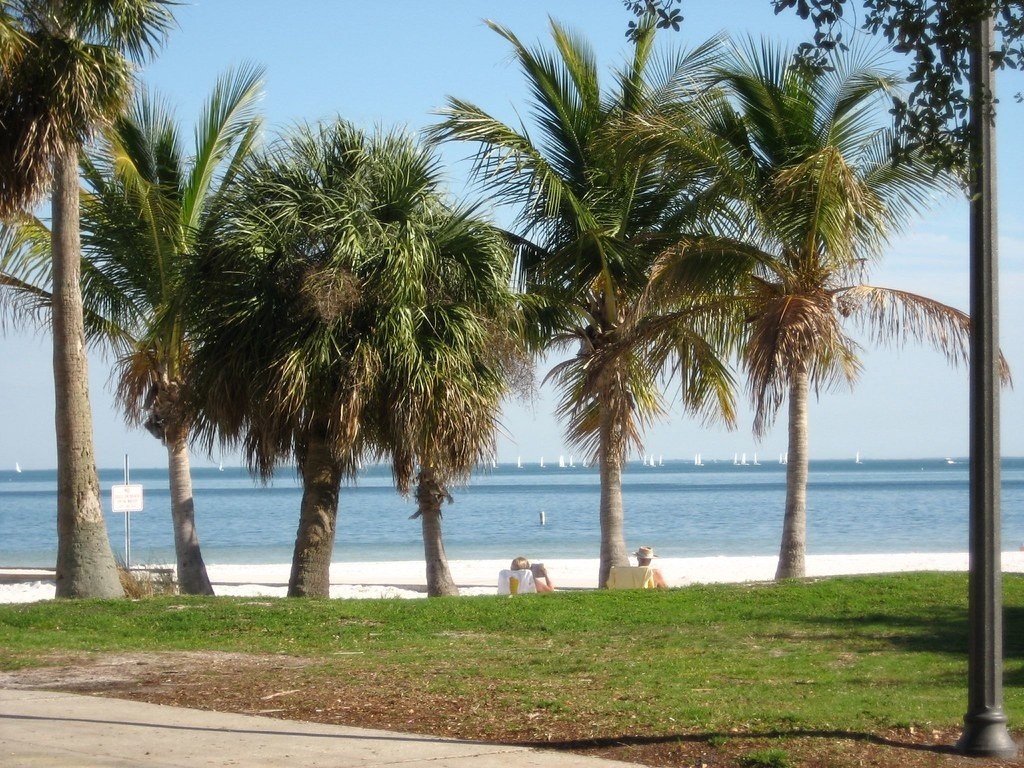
[531,564,544,577]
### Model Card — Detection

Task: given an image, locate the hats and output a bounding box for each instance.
[631,546,659,559]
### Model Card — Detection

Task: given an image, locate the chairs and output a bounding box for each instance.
[498,569,538,596]
[608,565,655,590]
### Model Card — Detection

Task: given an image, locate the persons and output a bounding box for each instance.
[632,547,668,590]
[510,556,554,593]
